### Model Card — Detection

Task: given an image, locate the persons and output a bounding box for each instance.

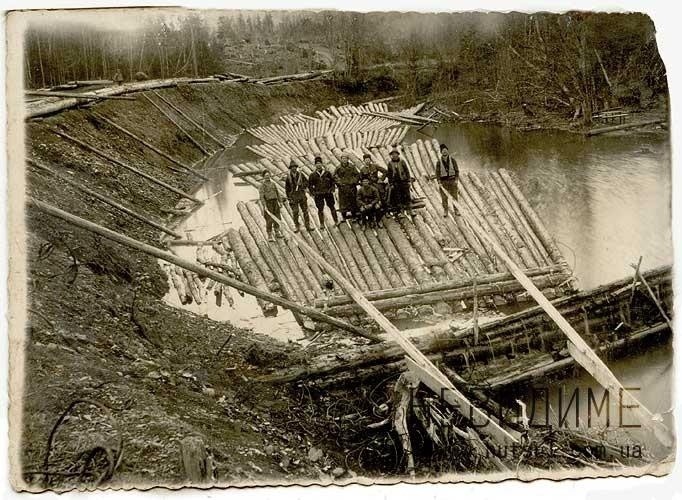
[309,155,340,229]
[259,169,285,243]
[285,159,316,233]
[332,152,360,223]
[433,144,461,218]
[389,147,418,220]
[360,153,388,182]
[356,178,381,232]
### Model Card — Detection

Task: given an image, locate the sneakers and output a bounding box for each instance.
[361,212,390,231]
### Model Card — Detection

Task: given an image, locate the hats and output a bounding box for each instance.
[389,150,400,155]
[440,144,448,153]
[363,154,370,158]
[315,157,322,164]
[288,160,298,169]
[341,153,349,159]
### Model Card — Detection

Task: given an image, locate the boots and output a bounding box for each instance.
[351,214,357,223]
[268,228,284,242]
[293,214,346,233]
[409,207,416,216]
[443,208,461,217]
